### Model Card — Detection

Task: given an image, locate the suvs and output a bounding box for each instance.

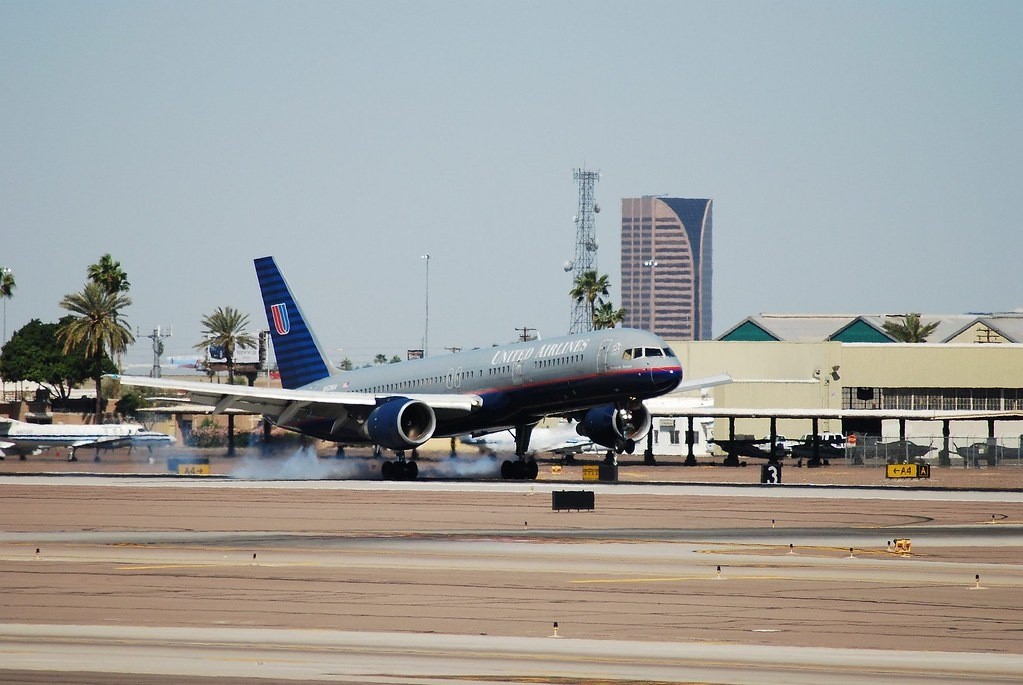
[800,433,848,449]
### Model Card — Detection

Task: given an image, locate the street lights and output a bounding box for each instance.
[3,266,13,345]
[422,252,430,357]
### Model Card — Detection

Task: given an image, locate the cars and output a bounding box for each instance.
[755,434,794,451]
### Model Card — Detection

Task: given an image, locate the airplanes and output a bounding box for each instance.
[105,255,819,479]
[0,412,175,463]
[702,431,1023,467]
